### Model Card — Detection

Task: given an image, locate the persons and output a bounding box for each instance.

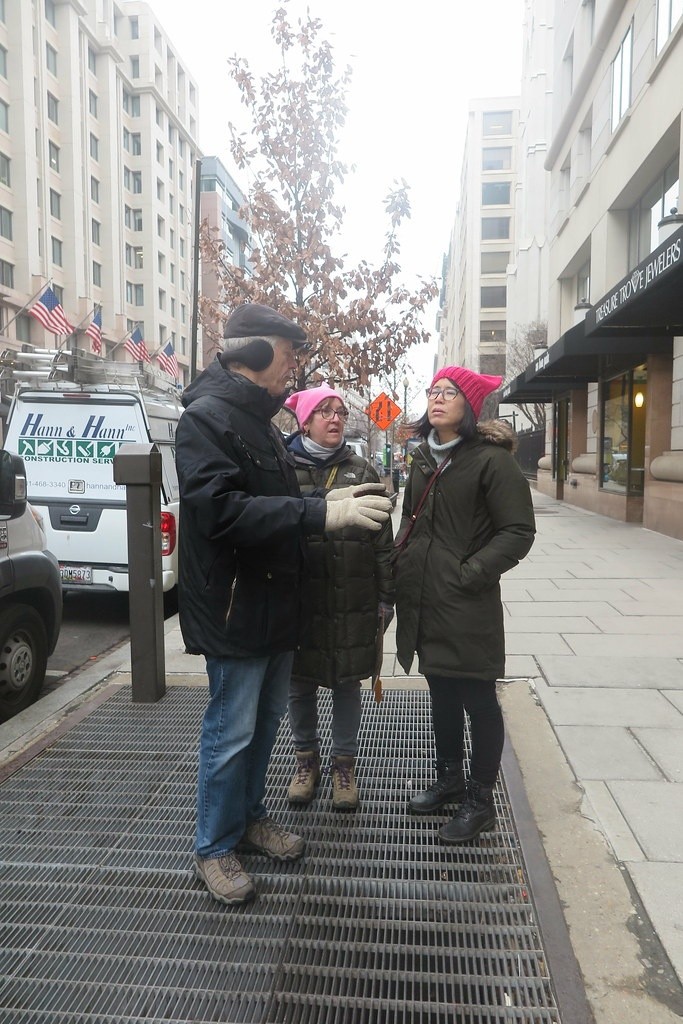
[174,301,393,905]
[390,365,538,846]
[282,388,393,808]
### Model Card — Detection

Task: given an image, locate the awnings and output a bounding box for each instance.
[489,228,683,404]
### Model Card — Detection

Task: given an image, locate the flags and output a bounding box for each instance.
[124,327,152,365]
[157,342,180,381]
[28,287,76,336]
[85,311,102,353]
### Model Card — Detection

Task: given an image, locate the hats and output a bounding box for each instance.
[223,303,306,349]
[284,387,344,433]
[429,366,503,419]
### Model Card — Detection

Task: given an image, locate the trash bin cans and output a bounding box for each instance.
[384,468,399,494]
[603,437,613,465]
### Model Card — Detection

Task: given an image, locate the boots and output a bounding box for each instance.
[408,761,466,812]
[438,776,494,842]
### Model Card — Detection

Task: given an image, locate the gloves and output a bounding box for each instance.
[324,483,391,501]
[323,494,392,533]
[378,602,394,633]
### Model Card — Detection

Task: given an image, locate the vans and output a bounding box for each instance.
[345,442,384,466]
[0,347,185,596]
[0,449,63,725]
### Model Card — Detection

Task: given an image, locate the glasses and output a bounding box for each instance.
[312,408,349,422]
[426,387,464,401]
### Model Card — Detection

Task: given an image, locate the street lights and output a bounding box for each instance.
[403,377,409,465]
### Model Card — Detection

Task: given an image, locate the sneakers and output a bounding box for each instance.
[235,814,305,861]
[195,852,257,904]
[289,751,320,803]
[330,756,359,809]
